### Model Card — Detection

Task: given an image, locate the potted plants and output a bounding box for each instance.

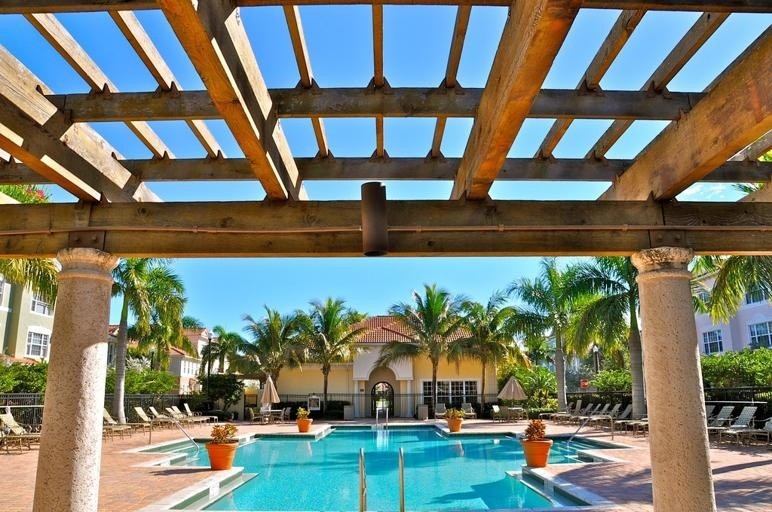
[520,418,554,468]
[295,407,314,432]
[204,424,242,471]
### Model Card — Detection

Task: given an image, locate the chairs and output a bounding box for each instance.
[705,405,772,449]
[247,406,293,423]
[102,403,220,442]
[417,399,647,435]
[0,413,41,452]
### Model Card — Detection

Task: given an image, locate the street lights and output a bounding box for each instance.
[205,330,215,414]
[592,341,599,374]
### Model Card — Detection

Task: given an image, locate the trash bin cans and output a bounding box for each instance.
[417,404,430,420]
[343,405,355,420]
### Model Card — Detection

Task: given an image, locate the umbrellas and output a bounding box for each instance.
[260,375,280,424]
[497,376,528,408]
